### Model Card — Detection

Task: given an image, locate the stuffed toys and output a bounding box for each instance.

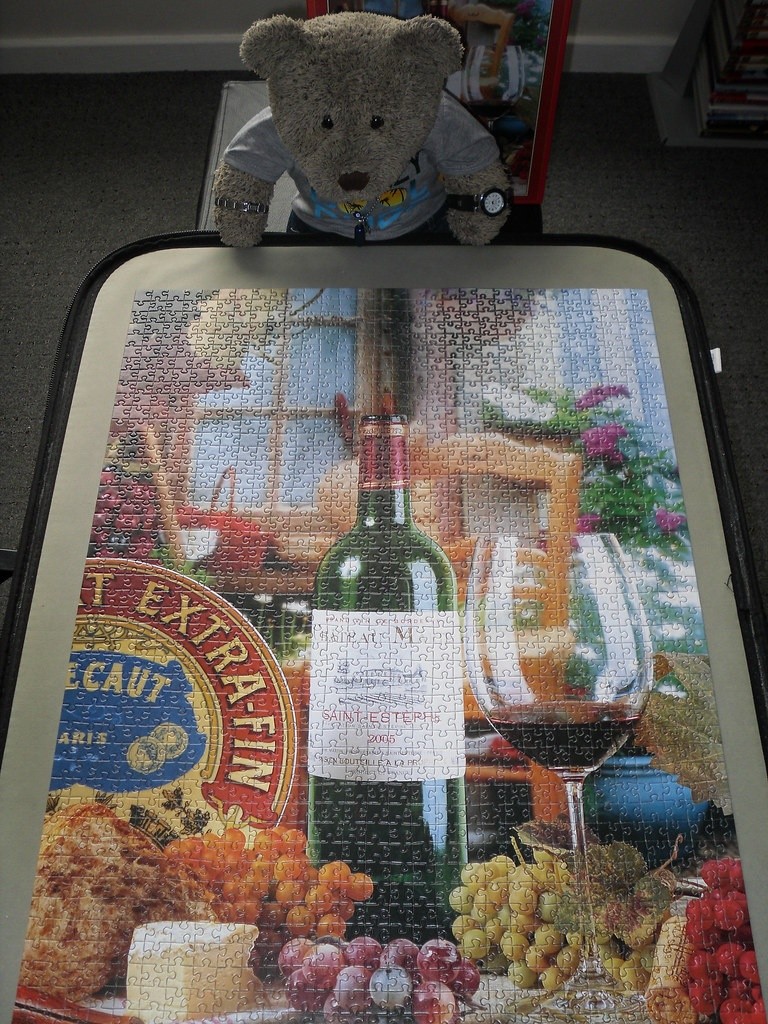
[211,11,511,247]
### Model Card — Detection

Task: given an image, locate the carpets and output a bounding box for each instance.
[192,78,298,235]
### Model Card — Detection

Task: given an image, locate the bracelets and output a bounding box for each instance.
[214,198,269,214]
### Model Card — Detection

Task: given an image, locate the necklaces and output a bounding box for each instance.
[348,196,381,240]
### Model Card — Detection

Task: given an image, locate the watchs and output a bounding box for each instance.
[447,188,507,217]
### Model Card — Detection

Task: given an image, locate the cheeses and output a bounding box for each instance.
[126,919,259,1022]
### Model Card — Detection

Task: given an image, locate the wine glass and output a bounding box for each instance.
[466,533,664,1022]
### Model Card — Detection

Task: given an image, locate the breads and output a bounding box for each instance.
[16,800,219,1002]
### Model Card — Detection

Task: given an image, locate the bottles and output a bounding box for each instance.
[309,414,466,942]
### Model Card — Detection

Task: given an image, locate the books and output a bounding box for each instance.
[693,0,768,137]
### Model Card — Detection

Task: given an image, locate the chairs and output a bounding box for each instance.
[304,0,573,234]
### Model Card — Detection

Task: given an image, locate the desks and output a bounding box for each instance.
[1,233,767,1022]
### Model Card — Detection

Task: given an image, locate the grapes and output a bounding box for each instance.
[165,826,766,1024]
